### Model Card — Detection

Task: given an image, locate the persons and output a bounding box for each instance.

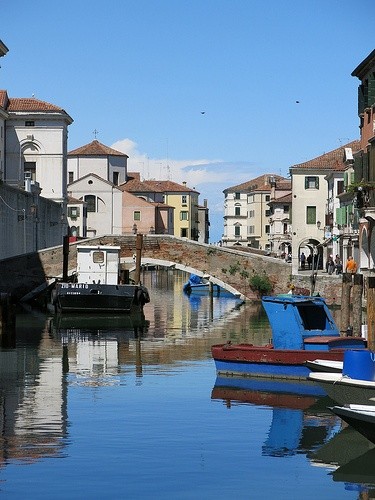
[327,257,334,275]
[149,226,156,234]
[133,224,137,234]
[334,255,342,275]
[218,240,223,247]
[314,254,318,269]
[234,240,242,246]
[307,255,312,270]
[346,256,358,274]
[301,253,306,270]
[276,248,292,262]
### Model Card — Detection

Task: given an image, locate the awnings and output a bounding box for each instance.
[316,238,331,248]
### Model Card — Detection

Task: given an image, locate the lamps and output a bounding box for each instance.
[317,221,325,230]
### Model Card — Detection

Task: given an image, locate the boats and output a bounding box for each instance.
[311,422,375,500]
[210,245,375,439]
[182,274,239,300]
[50,233,151,330]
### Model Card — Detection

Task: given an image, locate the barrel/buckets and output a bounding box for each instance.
[342,350,375,381]
